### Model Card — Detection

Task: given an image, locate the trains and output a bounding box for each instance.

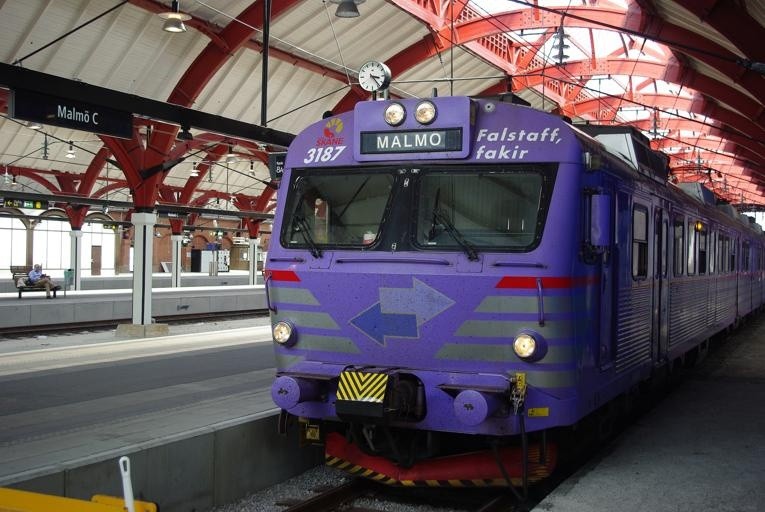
[265,96,765,489]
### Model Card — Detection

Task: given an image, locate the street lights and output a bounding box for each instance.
[13,0,193,68]
[261,0,360,126]
[5,140,77,167]
[192,160,278,191]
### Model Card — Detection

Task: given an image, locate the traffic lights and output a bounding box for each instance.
[104,225,116,230]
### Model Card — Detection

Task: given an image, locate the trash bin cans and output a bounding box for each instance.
[64,269,74,285]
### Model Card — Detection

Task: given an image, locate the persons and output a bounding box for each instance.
[29,264,61,300]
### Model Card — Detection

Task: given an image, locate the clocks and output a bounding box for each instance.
[359,62,390,92]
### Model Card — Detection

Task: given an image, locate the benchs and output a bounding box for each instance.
[10,266,61,299]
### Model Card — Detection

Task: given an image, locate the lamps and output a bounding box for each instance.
[65,143,76,159]
[189,162,201,177]
[157,0,192,33]
[225,142,237,164]
[12,175,18,185]
[330,0,366,19]
[26,120,42,129]
